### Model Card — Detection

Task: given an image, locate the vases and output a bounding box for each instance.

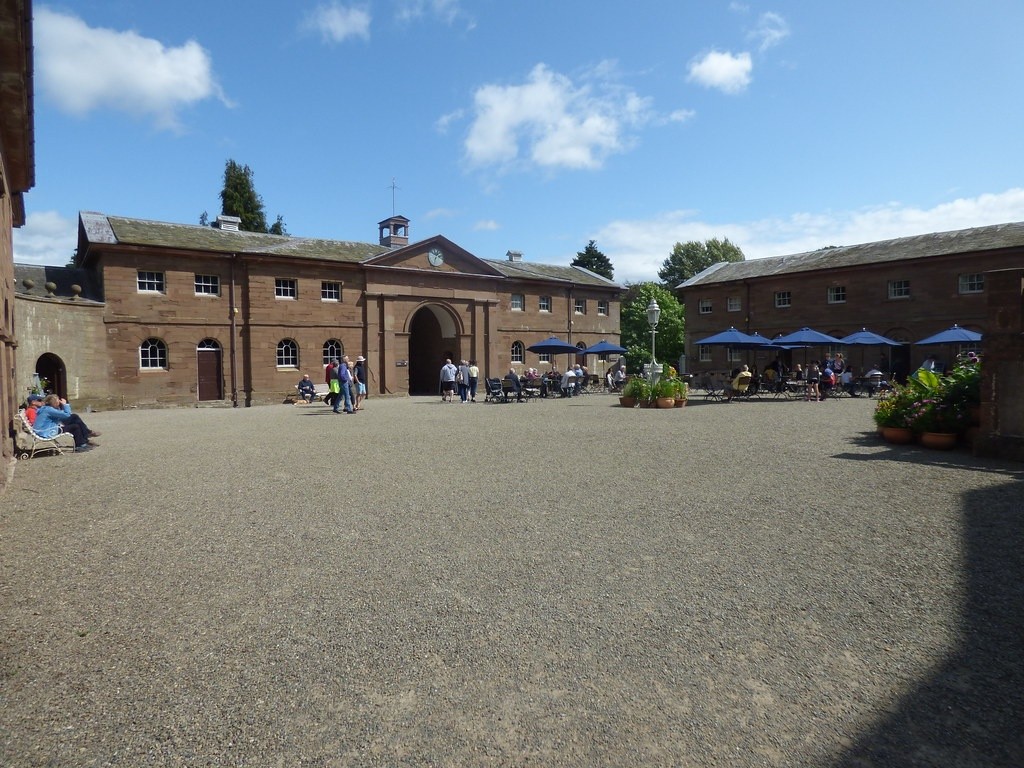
[877,425,913,444]
[919,430,958,449]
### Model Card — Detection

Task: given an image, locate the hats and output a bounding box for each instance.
[27,394,45,403]
[356,355,366,361]
[741,364,749,370]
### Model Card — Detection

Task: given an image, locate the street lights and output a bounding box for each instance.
[647,298,661,390]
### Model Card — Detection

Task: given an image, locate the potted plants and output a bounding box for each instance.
[619,372,689,409]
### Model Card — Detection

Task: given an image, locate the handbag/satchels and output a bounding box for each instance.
[455,366,463,383]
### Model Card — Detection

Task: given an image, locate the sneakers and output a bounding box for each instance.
[87,429,101,438]
[75,443,93,453]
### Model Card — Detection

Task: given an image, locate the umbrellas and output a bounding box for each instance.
[693,327,846,376]
[839,327,905,372]
[577,339,628,378]
[525,335,583,371]
[913,324,983,354]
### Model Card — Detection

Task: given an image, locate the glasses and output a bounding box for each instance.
[34,399,42,402]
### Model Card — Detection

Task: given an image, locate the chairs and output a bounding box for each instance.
[485,374,621,404]
[694,372,883,404]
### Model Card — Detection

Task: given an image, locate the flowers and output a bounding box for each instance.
[873,352,981,433]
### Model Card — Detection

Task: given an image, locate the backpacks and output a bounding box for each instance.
[808,366,820,382]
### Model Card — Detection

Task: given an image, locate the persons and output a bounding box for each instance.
[922,356,936,369]
[26,394,101,452]
[440,359,457,402]
[322,355,358,414]
[504,368,524,403]
[724,353,884,403]
[353,356,366,410]
[606,364,626,392]
[458,359,479,403]
[521,364,590,398]
[298,374,316,403]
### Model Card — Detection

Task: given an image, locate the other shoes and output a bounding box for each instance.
[333,409,341,414]
[804,399,810,402]
[449,400,452,403]
[353,407,364,410]
[323,398,329,406]
[727,397,732,401]
[470,398,475,402]
[462,400,467,404]
[815,398,819,401]
[346,411,357,414]
[441,398,446,402]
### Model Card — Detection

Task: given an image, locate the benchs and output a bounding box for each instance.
[13,408,75,461]
[294,384,330,402]
